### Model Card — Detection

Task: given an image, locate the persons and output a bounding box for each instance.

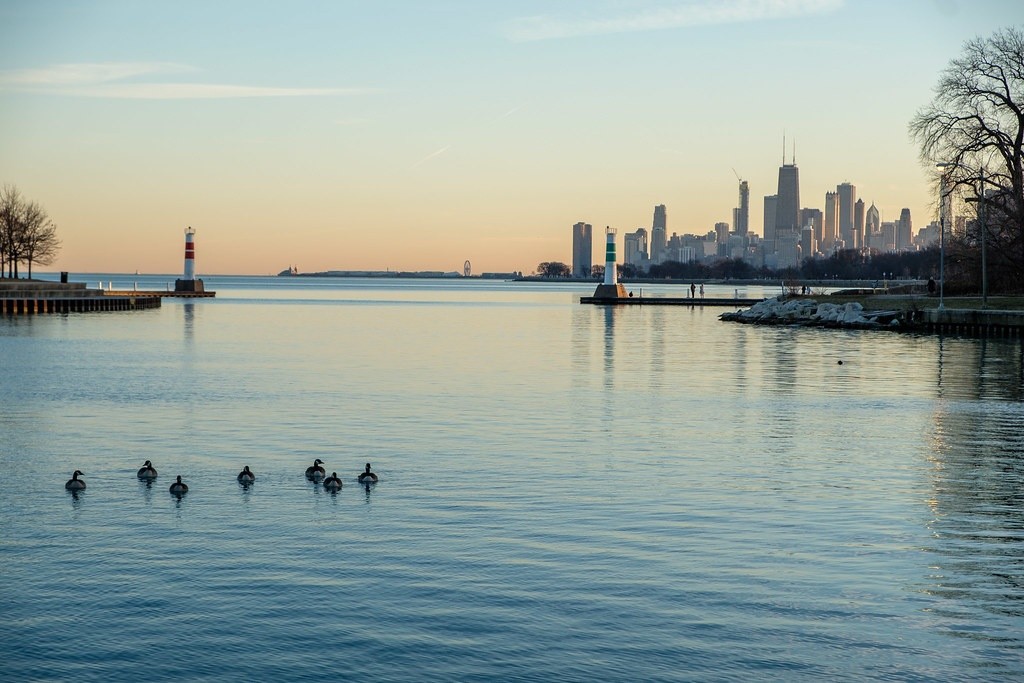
[802,285,811,294]
[926,275,936,295]
[700,283,705,298]
[691,282,696,298]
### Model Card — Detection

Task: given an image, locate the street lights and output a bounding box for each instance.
[937,162,987,308]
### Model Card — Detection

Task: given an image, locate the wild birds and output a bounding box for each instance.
[305,459,325,476]
[65,470,86,489]
[170,474,188,491]
[323,472,342,486]
[138,460,157,475]
[237,466,255,480]
[358,463,378,481]
